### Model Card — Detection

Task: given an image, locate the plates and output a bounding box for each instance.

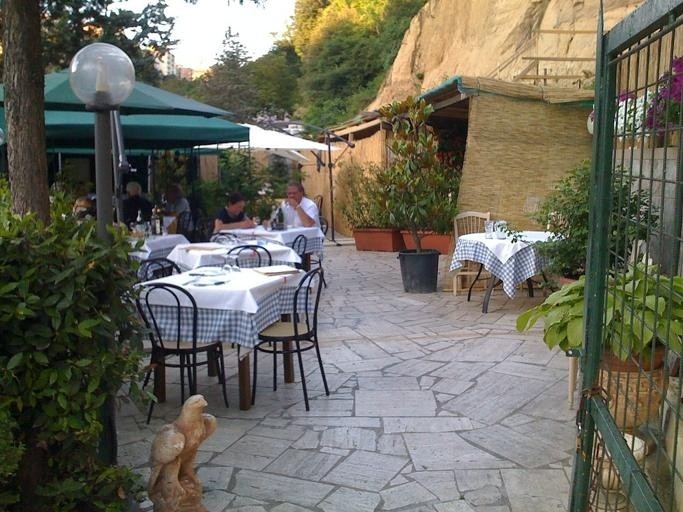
[188,269,226,276]
[193,278,230,286]
[253,264,298,274]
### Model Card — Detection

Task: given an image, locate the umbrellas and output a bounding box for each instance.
[0,62,236,221]
[193,123,342,220]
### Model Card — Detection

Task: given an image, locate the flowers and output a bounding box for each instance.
[585,57,683,147]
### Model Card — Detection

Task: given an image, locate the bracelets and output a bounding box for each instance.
[293,205,301,211]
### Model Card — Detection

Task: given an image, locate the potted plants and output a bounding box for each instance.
[534,161,658,286]
[383,155,453,255]
[336,151,404,251]
[374,96,464,294]
[513,262,683,430]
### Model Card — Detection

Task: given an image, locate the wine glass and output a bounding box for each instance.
[231,255,240,272]
[253,216,260,231]
[222,254,231,273]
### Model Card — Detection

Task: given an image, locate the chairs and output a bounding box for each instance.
[453,211,491,297]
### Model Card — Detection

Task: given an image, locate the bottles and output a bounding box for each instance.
[135,210,145,231]
[151,208,161,236]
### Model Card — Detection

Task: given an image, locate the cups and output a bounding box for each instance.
[494,220,507,239]
[162,225,168,237]
[484,221,493,239]
[257,237,267,246]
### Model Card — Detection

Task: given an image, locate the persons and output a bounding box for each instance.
[76,180,209,243]
[261,181,321,272]
[210,191,257,243]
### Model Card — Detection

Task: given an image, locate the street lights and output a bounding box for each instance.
[67,42,134,269]
